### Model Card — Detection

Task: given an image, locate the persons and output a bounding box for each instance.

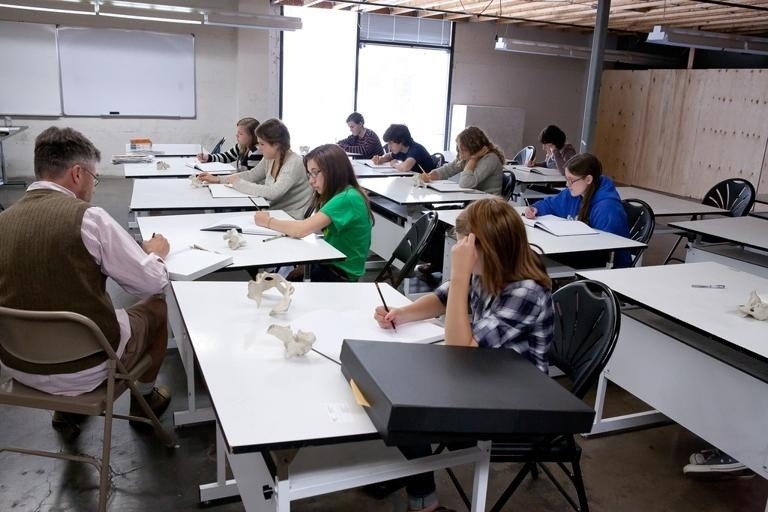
[197,119,315,218]
[372,123,435,177]
[416,124,506,275]
[253,145,376,282]
[196,117,268,180]
[524,153,633,268]
[523,124,577,208]
[0,127,171,430]
[335,112,385,161]
[357,198,578,510]
[682,440,757,483]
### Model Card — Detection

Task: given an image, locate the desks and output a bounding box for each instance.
[0,127,27,189]
[574,262,768,482]
[667,216,767,282]
[171,280,492,511]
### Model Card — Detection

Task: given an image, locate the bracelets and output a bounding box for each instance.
[267,217,275,229]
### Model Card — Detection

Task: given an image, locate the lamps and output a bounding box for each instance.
[497,1,669,65]
[98,0,203,24]
[203,1,302,31]
[646,0,767,55]
[0,0,98,18]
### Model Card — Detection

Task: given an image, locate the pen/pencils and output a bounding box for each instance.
[533,156,536,163]
[418,164,432,182]
[263,234,282,242]
[248,196,260,211]
[691,285,725,288]
[201,145,203,159]
[525,198,536,218]
[152,233,155,238]
[377,155,378,165]
[375,280,397,332]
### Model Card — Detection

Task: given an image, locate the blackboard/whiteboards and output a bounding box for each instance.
[0,20,63,117]
[56,24,197,119]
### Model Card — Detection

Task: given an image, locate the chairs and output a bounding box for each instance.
[0,307,172,512]
[433,281,621,509]
[664,178,756,265]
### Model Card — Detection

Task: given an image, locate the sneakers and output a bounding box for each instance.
[52,411,83,428]
[129,386,170,423]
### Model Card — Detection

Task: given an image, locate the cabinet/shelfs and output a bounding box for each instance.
[450,104,525,160]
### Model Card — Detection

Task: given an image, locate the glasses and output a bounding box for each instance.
[565,176,584,185]
[305,170,322,178]
[79,164,101,186]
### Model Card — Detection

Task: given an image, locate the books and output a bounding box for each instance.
[519,213,597,237]
[416,176,474,193]
[142,228,231,282]
[288,304,453,363]
[516,164,563,175]
[207,181,255,198]
[185,158,236,173]
[198,213,291,236]
[357,157,393,168]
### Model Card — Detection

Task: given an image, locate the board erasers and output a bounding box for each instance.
[110,112,119,114]
